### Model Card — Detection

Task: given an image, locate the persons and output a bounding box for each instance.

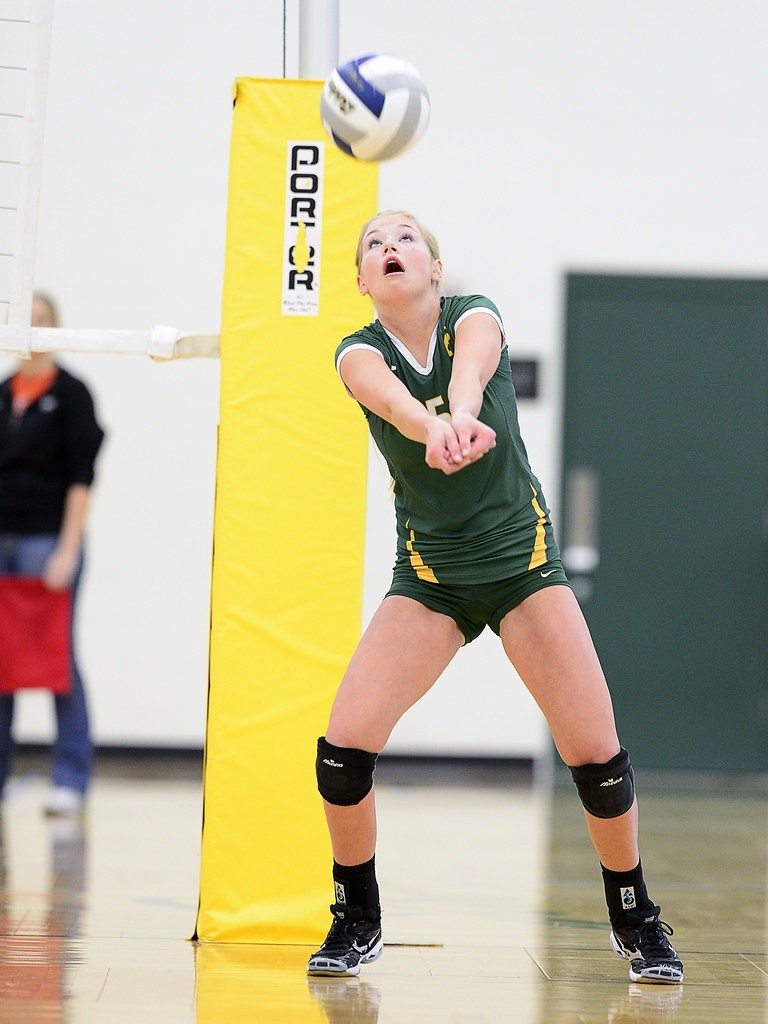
[306,212,688,987]
[0,287,106,819]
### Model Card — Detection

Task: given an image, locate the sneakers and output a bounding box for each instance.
[608,901,685,986]
[308,979,381,1024]
[307,904,387,976]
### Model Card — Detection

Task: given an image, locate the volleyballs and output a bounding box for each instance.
[321,47,434,167]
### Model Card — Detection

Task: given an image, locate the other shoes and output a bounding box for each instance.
[47,786,83,816]
